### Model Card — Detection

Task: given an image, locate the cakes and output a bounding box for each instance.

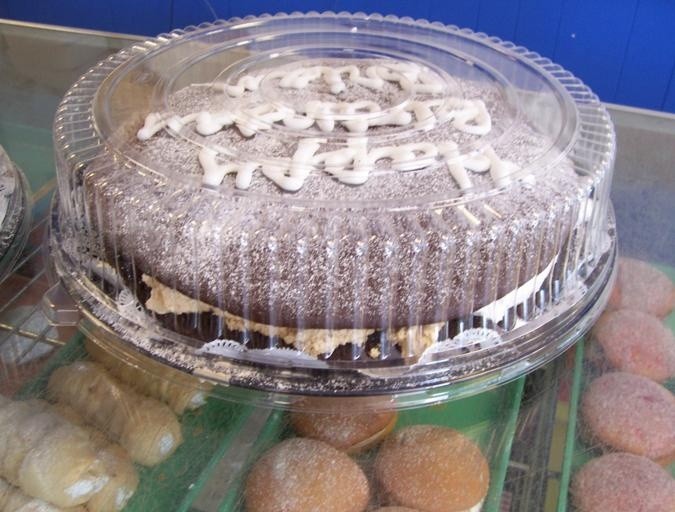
[0,143,23,277]
[80,59,585,387]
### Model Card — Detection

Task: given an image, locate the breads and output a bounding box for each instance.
[0,323,210,512]
[244,397,491,511]
[573,255,675,511]
